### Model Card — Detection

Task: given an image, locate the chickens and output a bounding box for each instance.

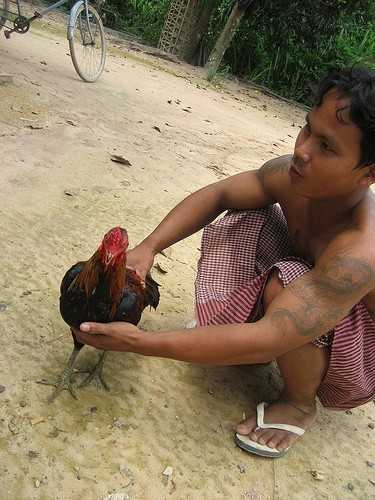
[35,226,162,405]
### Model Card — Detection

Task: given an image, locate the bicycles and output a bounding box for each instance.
[0,0,106,82]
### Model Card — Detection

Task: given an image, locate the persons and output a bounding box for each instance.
[70,61,374,459]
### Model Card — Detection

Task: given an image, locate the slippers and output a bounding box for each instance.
[235,402,306,458]
